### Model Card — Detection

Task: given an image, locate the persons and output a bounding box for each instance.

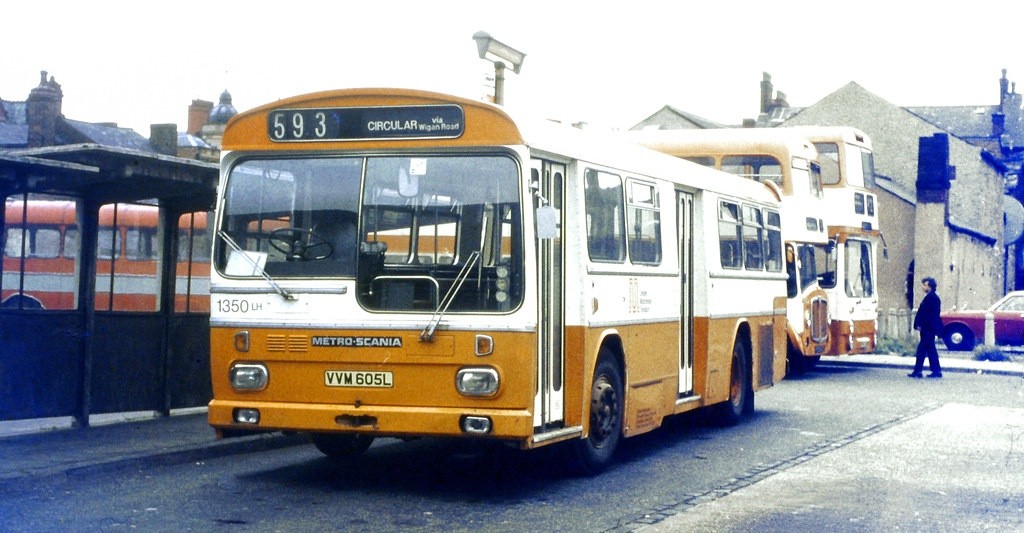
[907,277,944,378]
[785,244,797,298]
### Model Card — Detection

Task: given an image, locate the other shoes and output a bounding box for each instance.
[907,372,923,378]
[927,372,942,377]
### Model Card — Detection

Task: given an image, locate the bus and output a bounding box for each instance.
[370,125,891,379]
[208,86,789,479]
[0,198,290,314]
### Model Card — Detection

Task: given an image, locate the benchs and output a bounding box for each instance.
[381,262,502,312]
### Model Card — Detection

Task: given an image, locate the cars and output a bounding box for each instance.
[939,290,1024,351]
